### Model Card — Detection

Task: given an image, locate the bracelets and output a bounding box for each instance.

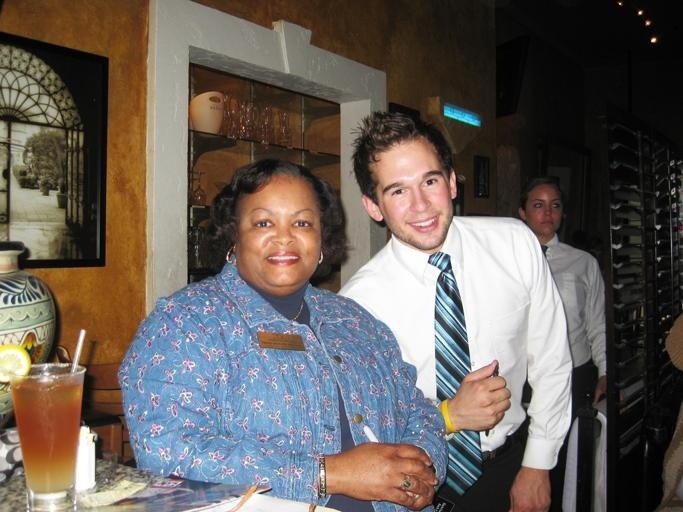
[318,452,327,499]
[441,398,462,433]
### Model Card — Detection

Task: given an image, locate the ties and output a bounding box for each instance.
[428,251,486,497]
[540,244,550,257]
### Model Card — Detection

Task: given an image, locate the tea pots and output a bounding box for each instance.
[223,91,292,146]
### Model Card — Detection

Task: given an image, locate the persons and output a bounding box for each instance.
[519,176,606,512]
[333,111,573,512]
[118,159,448,512]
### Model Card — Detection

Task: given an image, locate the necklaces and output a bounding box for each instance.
[256,299,305,351]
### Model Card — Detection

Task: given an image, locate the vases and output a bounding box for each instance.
[0,249,56,435]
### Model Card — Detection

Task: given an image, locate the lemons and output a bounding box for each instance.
[1,342,31,383]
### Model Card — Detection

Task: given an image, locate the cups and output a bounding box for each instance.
[9,364,85,511]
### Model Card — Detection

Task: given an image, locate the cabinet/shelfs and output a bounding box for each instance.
[188,62,340,293]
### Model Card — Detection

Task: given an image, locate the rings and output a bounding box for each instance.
[400,475,412,490]
